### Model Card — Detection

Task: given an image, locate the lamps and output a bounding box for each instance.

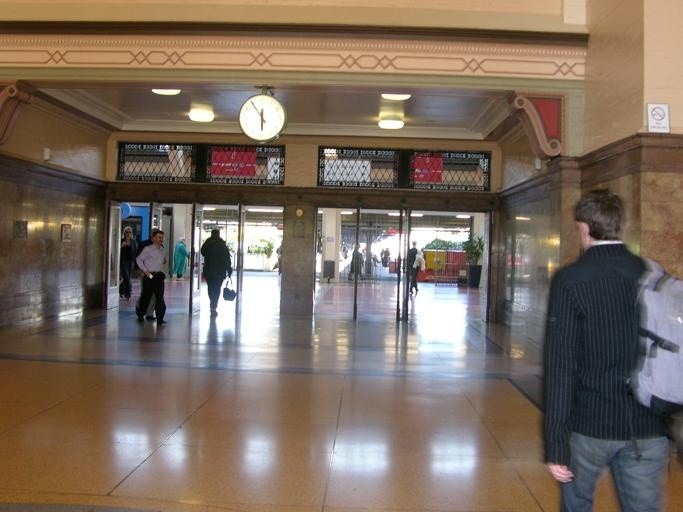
[378,113,405,129]
[188,103,216,122]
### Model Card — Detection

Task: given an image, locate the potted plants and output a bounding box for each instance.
[462,234,484,287]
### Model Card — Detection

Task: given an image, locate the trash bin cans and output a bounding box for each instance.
[324,260,335,278]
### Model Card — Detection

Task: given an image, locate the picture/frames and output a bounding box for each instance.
[61,223,72,242]
[13,219,29,240]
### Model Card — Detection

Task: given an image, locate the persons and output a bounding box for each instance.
[407,241,420,293]
[172,237,190,282]
[371,247,391,267]
[541,188,682,511]
[135,230,169,326]
[347,243,365,281]
[118,226,138,300]
[200,229,232,316]
[133,228,165,321]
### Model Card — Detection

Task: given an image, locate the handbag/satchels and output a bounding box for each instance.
[223,288,236,301]
[631,260,683,406]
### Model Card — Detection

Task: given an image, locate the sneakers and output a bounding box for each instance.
[138,315,167,325]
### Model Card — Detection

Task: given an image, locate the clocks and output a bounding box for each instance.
[237,83,288,143]
